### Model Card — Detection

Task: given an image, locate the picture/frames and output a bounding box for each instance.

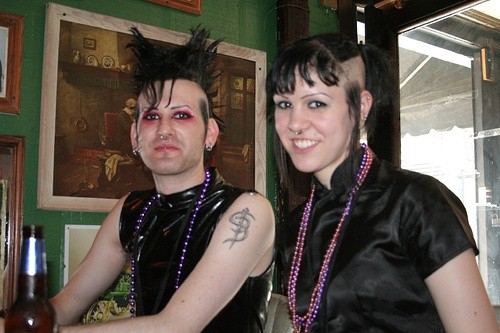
[0,133,27,319]
[36,1,269,215]
[63,224,137,325]
[0,11,26,115]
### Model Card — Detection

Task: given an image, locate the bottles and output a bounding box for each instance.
[5,224,59,333]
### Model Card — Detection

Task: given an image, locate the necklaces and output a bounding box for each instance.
[129,169,210,319]
[287,143,375,333]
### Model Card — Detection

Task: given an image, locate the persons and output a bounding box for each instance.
[265,32,499,333]
[0,22,276,333]
